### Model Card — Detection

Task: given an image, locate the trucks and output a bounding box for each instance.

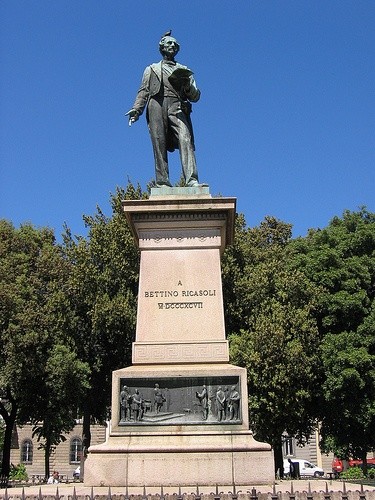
[330,455,375,479]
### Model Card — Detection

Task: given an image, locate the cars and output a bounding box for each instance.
[71,466,81,480]
[280,458,325,477]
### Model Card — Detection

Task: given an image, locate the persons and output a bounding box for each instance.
[288,459,295,478]
[120,383,166,422]
[125,30,209,188]
[47,472,61,484]
[195,385,240,420]
[332,454,343,472]
